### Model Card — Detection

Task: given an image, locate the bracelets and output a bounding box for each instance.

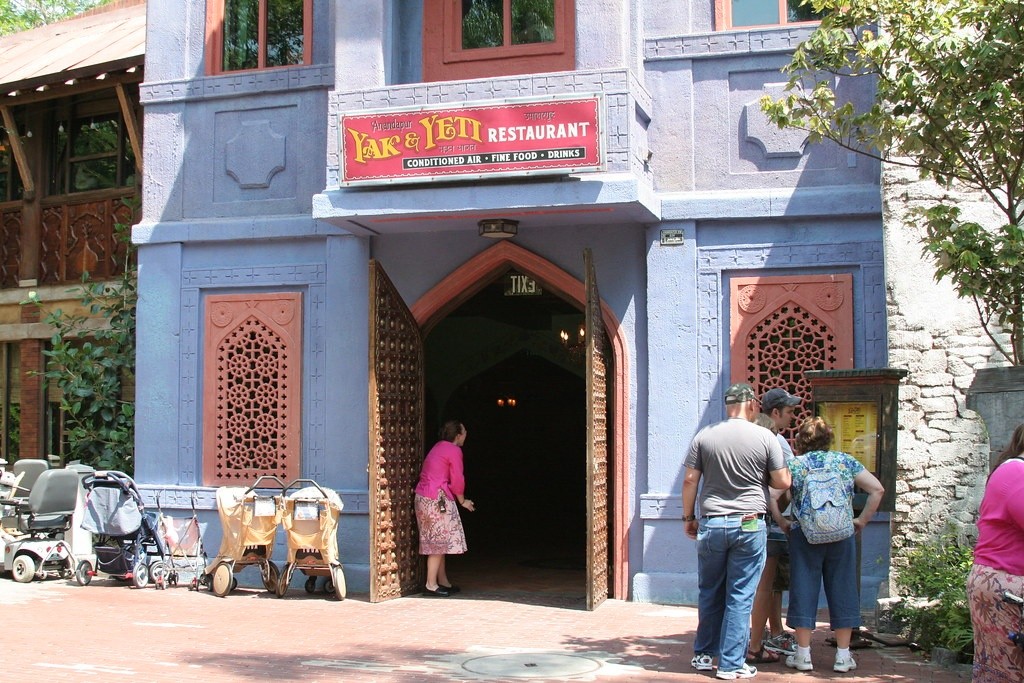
[682,515,695,521]
[461,501,464,505]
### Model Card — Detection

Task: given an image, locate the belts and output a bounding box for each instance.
[702,513,767,520]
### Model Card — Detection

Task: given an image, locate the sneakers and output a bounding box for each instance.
[785,652,813,670]
[716,663,757,680]
[690,653,713,670]
[833,650,857,672]
[763,631,799,655]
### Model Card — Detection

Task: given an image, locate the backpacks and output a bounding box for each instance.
[792,450,855,544]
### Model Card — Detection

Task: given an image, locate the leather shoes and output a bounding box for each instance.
[423,585,449,597]
[438,583,460,593]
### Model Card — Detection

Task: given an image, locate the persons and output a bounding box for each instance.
[744,389,802,665]
[767,415,884,672]
[682,383,791,680]
[413,420,475,596]
[967,422,1024,683]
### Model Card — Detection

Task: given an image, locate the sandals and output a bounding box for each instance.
[746,643,779,664]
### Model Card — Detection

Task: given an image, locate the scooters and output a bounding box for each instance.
[0,458,78,583]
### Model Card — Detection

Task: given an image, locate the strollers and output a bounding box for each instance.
[204,475,285,597]
[276,478,347,600]
[75,470,170,589]
[155,490,208,591]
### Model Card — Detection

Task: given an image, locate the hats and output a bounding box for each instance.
[725,384,763,405]
[762,388,802,410]
